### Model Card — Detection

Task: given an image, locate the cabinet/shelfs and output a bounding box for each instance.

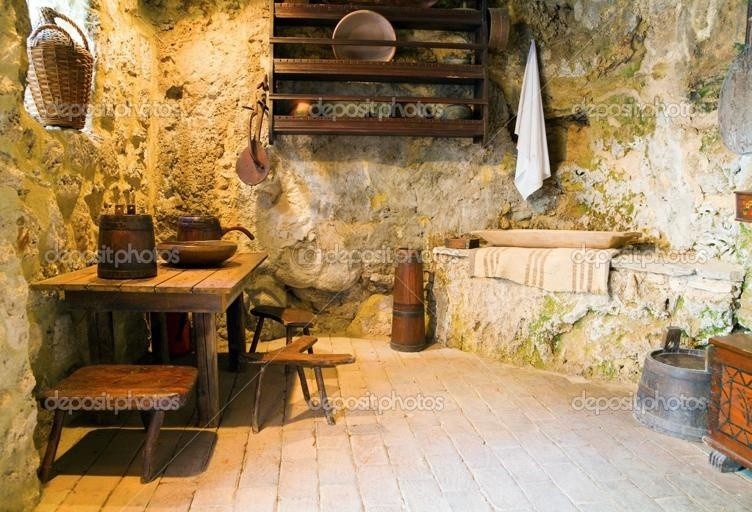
[703,334,752,468]
[268,1,490,144]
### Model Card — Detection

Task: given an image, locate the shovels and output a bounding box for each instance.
[236,93,270,186]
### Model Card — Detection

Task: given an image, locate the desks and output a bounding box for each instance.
[28,250,270,429]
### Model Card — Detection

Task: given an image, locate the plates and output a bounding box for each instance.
[428,34,472,63]
[441,104,472,120]
[331,9,397,65]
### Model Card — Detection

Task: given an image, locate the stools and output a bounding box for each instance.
[249,304,318,355]
[35,362,200,485]
[238,334,357,435]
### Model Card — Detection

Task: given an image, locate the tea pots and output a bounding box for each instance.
[176,215,254,249]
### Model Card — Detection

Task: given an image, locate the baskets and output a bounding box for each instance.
[25,7,94,130]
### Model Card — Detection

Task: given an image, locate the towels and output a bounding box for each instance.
[513,39,552,200]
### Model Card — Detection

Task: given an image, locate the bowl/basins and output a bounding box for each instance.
[158,241,237,268]
